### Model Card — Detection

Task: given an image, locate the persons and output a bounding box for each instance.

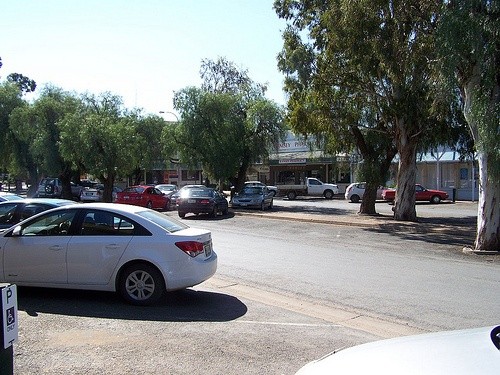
[298,176,304,185]
[340,175,344,181]
[139,175,144,185]
[205,178,210,187]
[316,176,320,181]
[154,174,158,184]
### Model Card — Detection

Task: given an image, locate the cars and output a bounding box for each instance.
[230,180,278,211]
[345,182,449,204]
[69,178,117,202]
[117,183,228,218]
[295,323,500,375]
[0,192,217,307]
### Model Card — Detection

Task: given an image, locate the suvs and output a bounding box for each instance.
[36,178,63,198]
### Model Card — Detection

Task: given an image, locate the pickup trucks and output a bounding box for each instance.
[276,177,338,200]
